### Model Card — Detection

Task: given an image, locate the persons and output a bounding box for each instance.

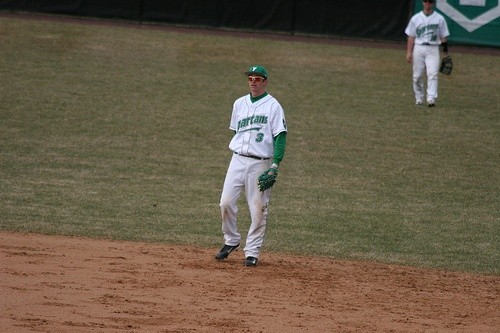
[214,65,287,267]
[404,0,451,107]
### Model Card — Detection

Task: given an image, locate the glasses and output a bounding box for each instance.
[248,76,265,82]
[422,0,433,4]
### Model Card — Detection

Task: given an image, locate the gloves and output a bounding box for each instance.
[258,168,278,191]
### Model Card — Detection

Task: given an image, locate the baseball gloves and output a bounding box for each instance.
[257,168,278,191]
[440,56,453,75]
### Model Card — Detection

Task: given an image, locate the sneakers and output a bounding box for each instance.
[215,243,240,259]
[243,257,258,266]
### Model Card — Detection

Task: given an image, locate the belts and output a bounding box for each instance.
[233,152,271,160]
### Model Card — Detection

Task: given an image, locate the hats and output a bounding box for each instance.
[244,64,269,78]
